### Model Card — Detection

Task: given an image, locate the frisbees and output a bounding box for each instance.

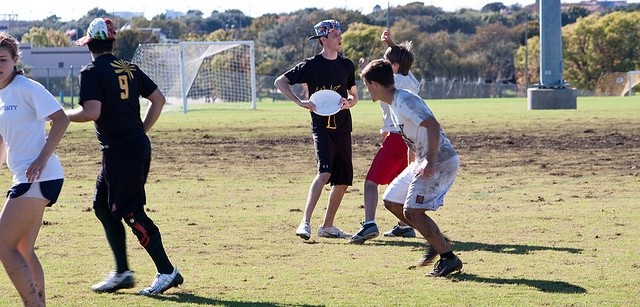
[309,89,344,116]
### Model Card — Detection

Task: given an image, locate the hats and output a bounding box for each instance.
[75,17,116,46]
[308,20,339,39]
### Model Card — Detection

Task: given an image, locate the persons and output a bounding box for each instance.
[50,16,184,295]
[349,28,422,246]
[0,32,71,307]
[274,19,358,241]
[360,59,463,278]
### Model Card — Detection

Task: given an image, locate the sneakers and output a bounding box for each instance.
[426,257,462,278]
[421,235,449,266]
[296,222,310,240]
[92,270,133,292]
[349,222,378,244]
[318,225,351,238]
[384,226,415,238]
[140,269,183,296]
